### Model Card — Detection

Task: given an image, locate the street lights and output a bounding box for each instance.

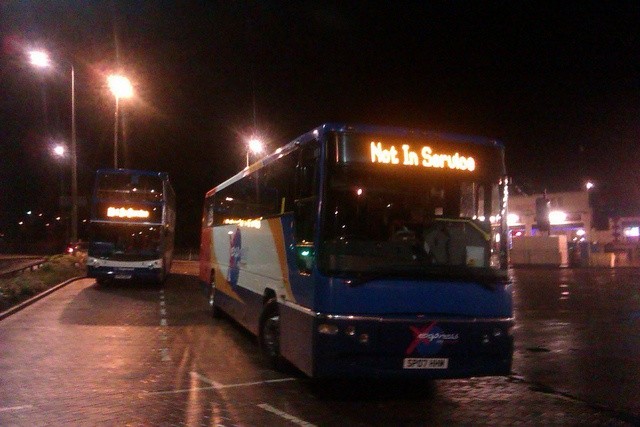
[242,131,265,166]
[29,46,77,249]
[104,72,136,166]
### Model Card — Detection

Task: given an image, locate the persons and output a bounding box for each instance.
[400,208,427,225]
[390,214,408,233]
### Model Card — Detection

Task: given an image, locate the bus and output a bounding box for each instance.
[198,121,551,380]
[84,165,177,284]
